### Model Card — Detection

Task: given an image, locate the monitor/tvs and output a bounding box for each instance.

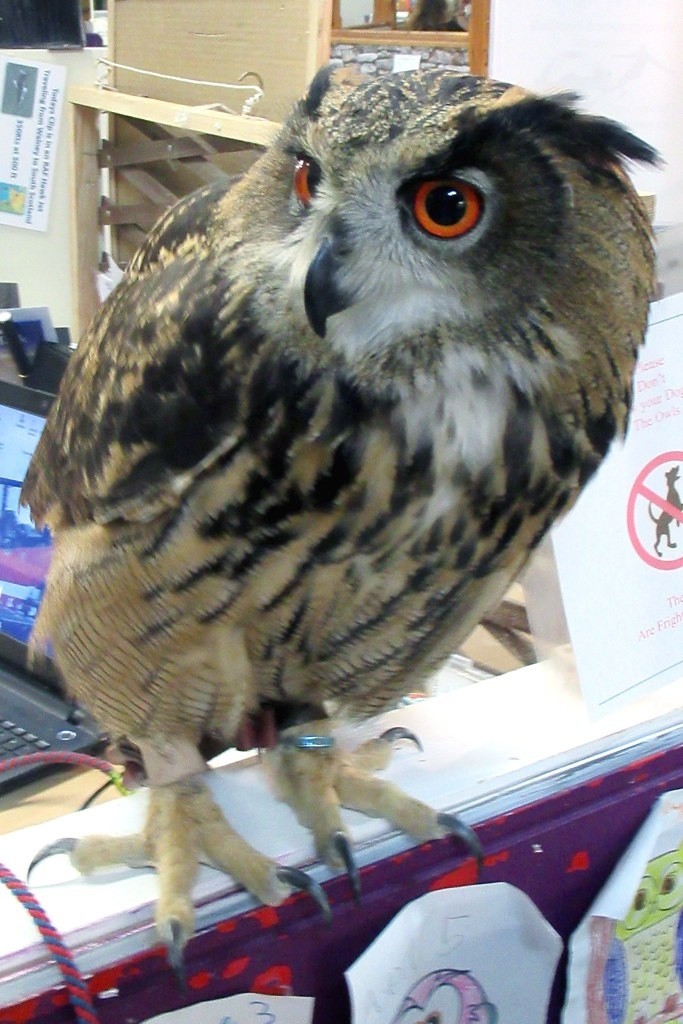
[0,0,86,49]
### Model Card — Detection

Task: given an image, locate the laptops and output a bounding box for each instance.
[1,379,116,794]
